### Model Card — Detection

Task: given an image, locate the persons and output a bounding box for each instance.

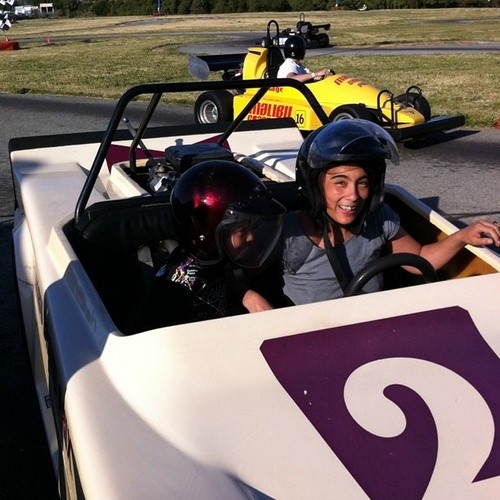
[106,160,287,334]
[277,36,331,82]
[230,119,500,313]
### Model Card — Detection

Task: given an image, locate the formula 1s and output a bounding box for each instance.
[260,13,331,59]
[2,79,500,500]
[189,46,466,149]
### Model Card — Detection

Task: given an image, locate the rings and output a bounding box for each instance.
[492,221,498,224]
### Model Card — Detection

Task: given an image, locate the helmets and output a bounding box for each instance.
[284,36,306,60]
[296,120,392,218]
[170,160,286,263]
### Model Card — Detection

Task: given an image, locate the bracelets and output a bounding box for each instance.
[310,72,316,78]
